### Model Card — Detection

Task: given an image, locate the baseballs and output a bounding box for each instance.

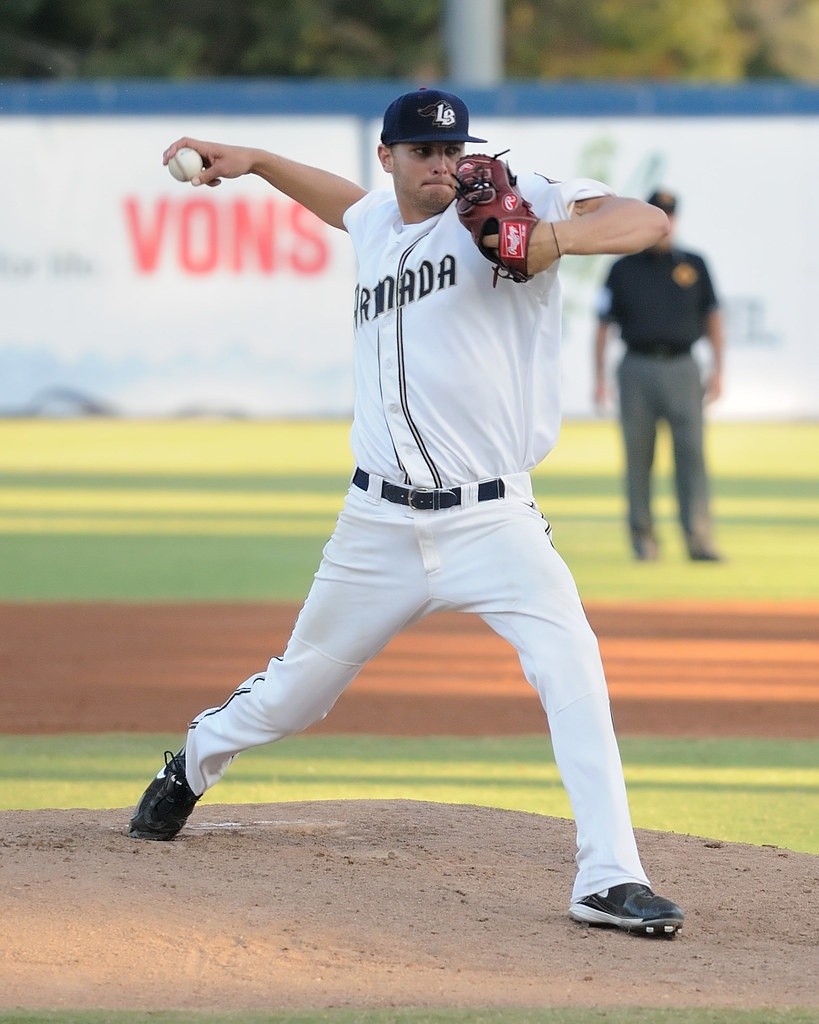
[168,147,204,182]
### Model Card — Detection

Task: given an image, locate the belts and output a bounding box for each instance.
[352,466,504,511]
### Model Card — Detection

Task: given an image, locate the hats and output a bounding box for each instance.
[647,192,676,209]
[381,88,488,144]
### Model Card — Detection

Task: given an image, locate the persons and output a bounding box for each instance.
[592,184,732,564]
[124,85,690,938]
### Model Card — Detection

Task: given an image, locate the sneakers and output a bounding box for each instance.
[128,744,202,841]
[568,883,684,935]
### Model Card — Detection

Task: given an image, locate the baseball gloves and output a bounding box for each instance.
[450,148,542,288]
